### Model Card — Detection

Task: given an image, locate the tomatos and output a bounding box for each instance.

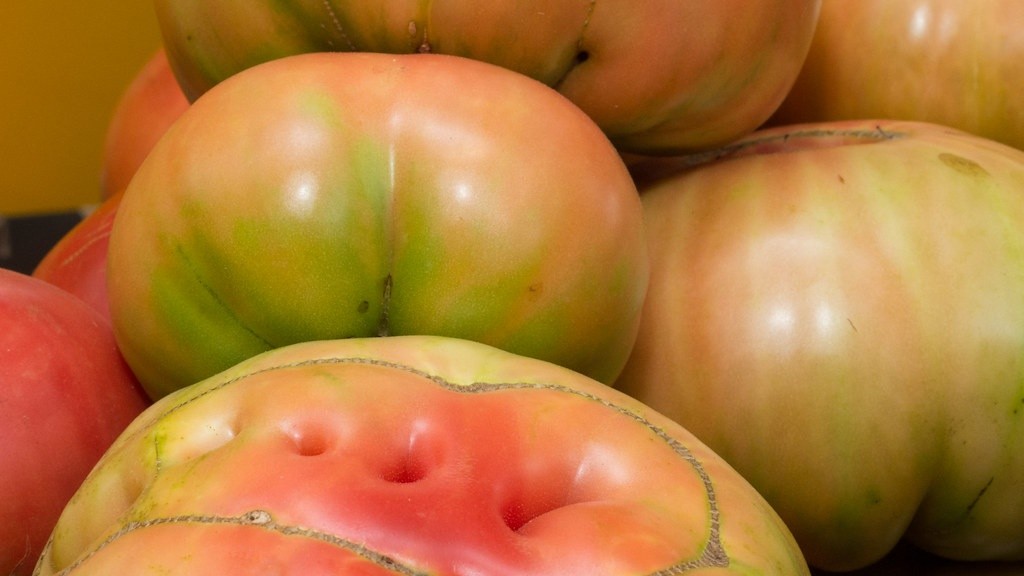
[0,0,1024,576]
